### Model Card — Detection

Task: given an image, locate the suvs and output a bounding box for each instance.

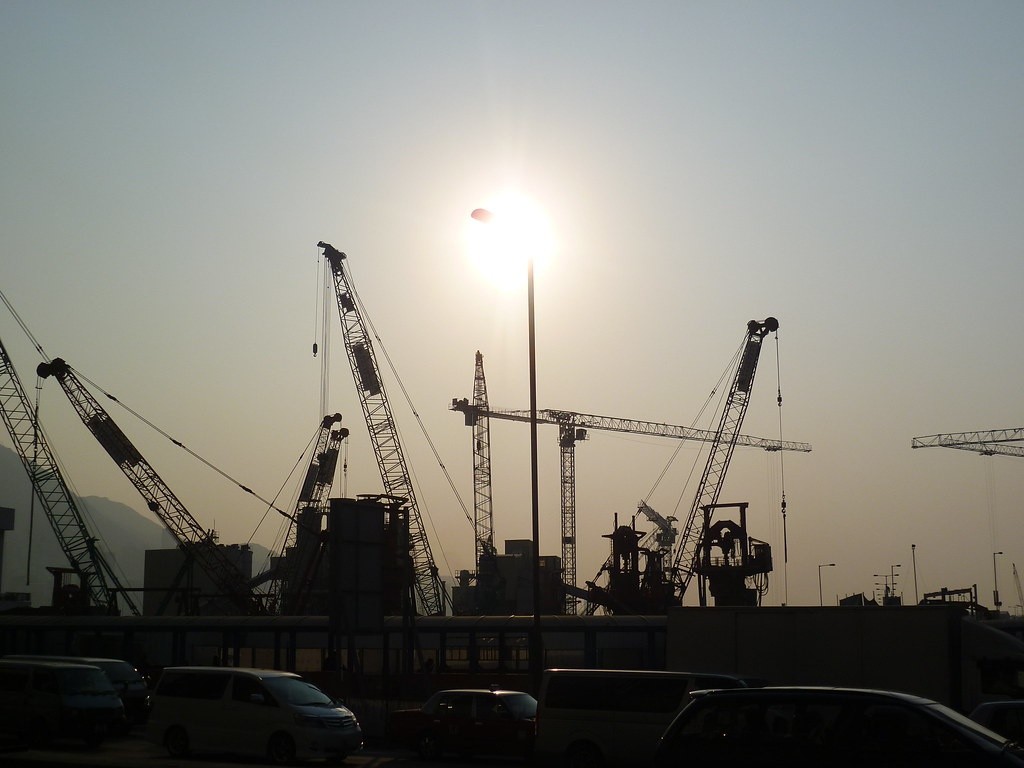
[649,687,1024,768]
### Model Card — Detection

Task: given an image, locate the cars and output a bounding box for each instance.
[3,654,147,737]
[386,688,539,760]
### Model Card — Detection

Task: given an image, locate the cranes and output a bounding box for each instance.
[461,349,504,616]
[264,412,350,615]
[36,358,265,614]
[0,339,118,613]
[668,317,778,602]
[546,408,585,617]
[317,241,449,617]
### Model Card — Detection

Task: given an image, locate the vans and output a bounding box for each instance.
[0,658,127,753]
[536,668,748,768]
[146,666,363,768]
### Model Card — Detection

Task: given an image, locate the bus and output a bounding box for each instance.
[0,615,666,746]
